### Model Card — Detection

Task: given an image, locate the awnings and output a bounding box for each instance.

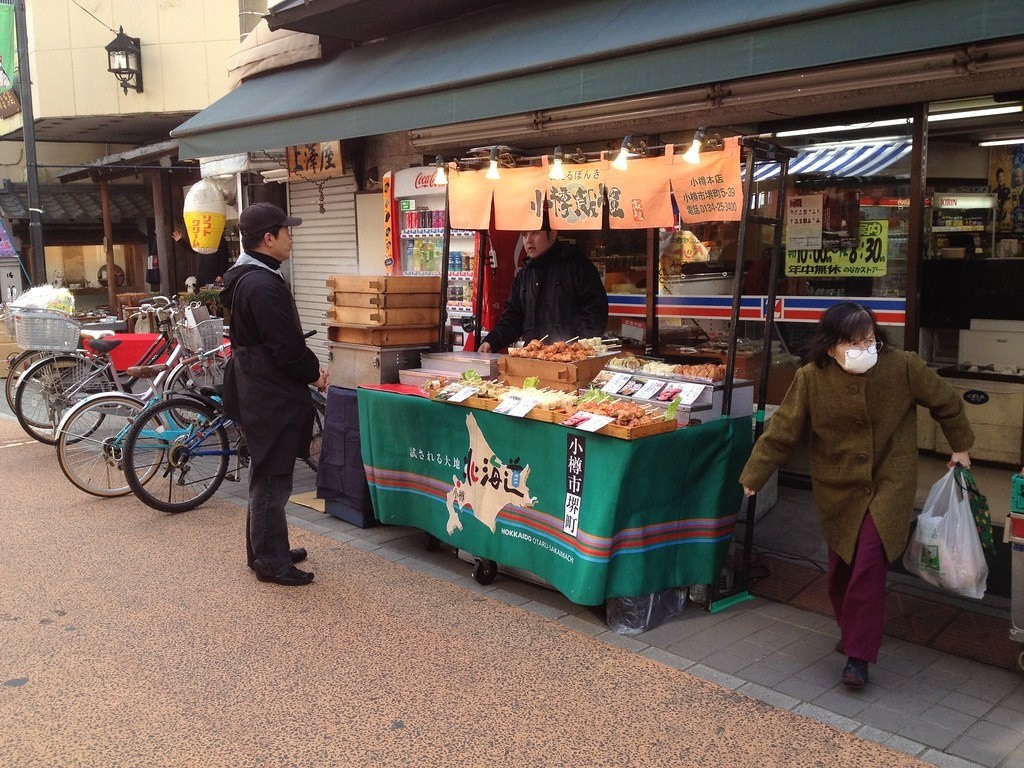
[169,0,1024,161]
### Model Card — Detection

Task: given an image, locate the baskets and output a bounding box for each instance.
[1010,473,1024,512]
[177,315,224,352]
[4,305,82,351]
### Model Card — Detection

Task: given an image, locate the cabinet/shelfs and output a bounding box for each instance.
[858,192,998,260]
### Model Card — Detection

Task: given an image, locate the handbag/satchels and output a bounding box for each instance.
[902,466,988,600]
[187,301,217,348]
[953,468,993,547]
[222,358,239,417]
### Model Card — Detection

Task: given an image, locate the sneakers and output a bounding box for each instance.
[842,657,868,688]
[836,639,844,653]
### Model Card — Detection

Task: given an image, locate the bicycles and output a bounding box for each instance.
[52,341,251,498]
[5,274,224,446]
[121,330,330,511]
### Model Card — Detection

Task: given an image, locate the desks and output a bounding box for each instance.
[82,333,177,373]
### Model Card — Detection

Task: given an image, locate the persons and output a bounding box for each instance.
[219,202,329,586]
[172,230,230,319]
[993,168,1024,221]
[919,207,981,367]
[739,304,977,689]
[477,211,608,353]
[742,247,774,295]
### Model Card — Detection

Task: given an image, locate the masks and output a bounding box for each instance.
[834,341,877,373]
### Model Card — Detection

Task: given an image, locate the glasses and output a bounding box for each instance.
[836,334,883,359]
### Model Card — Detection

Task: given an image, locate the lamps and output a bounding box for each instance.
[106,26,143,94]
[432,128,710,185]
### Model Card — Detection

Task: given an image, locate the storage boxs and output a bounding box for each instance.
[327,276,440,342]
[428,351,678,441]
[1011,474,1024,514]
[958,319,1024,370]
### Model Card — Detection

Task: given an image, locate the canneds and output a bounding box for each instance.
[449,286,473,302]
[403,209,445,229]
[449,250,474,271]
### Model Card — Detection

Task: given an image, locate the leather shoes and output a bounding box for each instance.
[248,547,307,572]
[256,566,314,586]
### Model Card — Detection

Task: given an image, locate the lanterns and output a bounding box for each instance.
[183,178,226,255]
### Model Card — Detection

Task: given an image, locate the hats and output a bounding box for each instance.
[761,247,778,257]
[238,202,302,236]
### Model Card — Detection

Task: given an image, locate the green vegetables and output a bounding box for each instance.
[663,396,681,421]
[522,376,540,389]
[576,384,614,405]
[459,369,481,380]
